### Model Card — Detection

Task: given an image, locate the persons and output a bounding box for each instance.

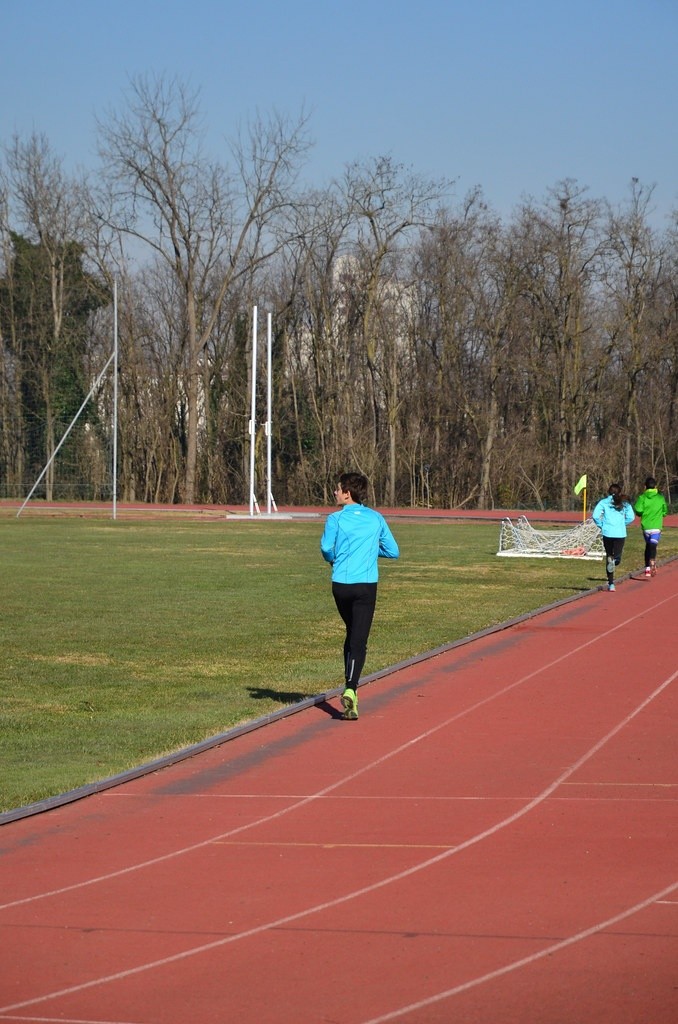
[592,483,636,591]
[633,477,668,578]
[319,473,400,722]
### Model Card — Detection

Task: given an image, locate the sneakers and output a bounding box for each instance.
[644,569,651,577]
[340,689,358,720]
[608,584,616,592]
[607,556,616,571]
[650,559,656,576]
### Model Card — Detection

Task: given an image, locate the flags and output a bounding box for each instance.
[574,475,587,496]
